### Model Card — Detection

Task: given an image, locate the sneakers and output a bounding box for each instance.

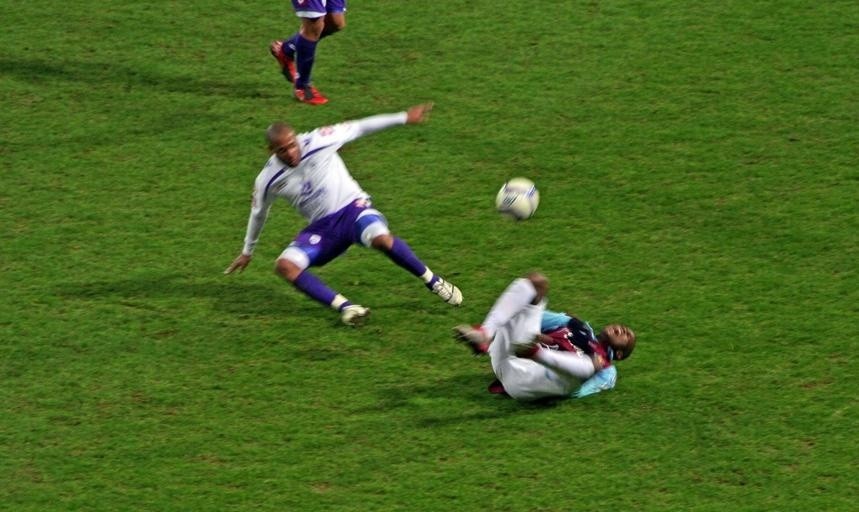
[339,304,373,327]
[450,326,490,355]
[431,275,464,307]
[293,85,329,106]
[270,39,298,84]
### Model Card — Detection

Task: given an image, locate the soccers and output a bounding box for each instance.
[497,177,539,220]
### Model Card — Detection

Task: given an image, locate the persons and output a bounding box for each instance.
[455,271,637,406]
[223,100,463,328]
[270,1,348,105]
